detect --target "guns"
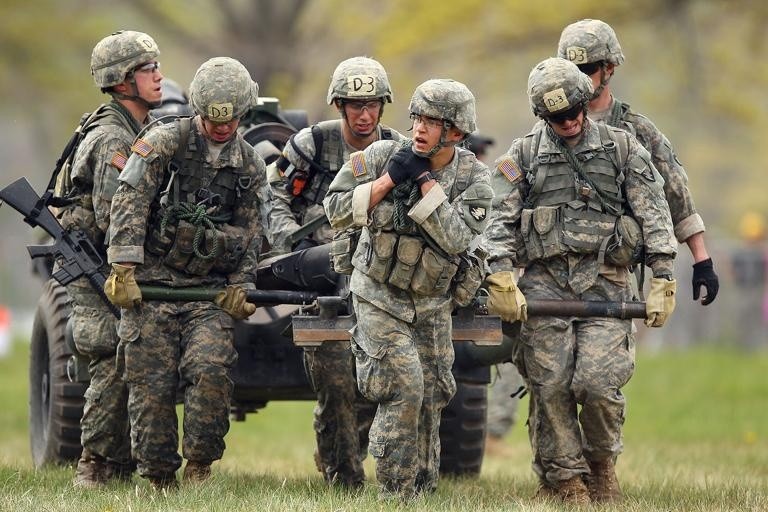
[1,177,120,320]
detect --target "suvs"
[28,77,517,487]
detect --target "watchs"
[657,274,674,281]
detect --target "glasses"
[580,63,606,75]
[547,106,581,124]
[134,61,162,75]
[411,115,444,130]
[344,99,381,113]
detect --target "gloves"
[103,265,142,310]
[213,285,256,320]
[645,276,677,329]
[692,259,720,307]
[387,145,430,185]
[485,271,527,322]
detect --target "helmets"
[327,58,393,104]
[189,57,260,122]
[526,58,592,119]
[558,19,625,67]
[91,29,159,89]
[410,79,477,134]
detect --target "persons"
[482,56,676,510]
[466,117,522,463]
[259,55,411,497]
[52,29,165,491]
[321,77,496,504]
[104,55,267,492]
[525,17,719,504]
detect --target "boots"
[150,459,212,488]
[532,460,620,505]
[75,446,135,490]
